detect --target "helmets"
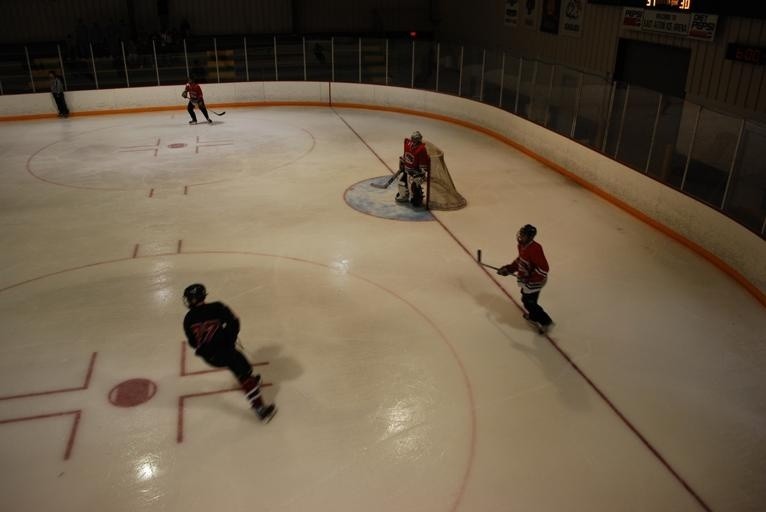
[183,284,206,308]
[411,131,422,141]
[516,224,536,244]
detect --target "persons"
[47,69,69,117]
[497,224,556,334]
[183,284,275,421]
[182,72,212,126]
[395,130,432,208]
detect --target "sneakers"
[189,118,212,123]
[256,404,274,420]
[523,313,553,334]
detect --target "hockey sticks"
[370,170,403,188]
[477,249,518,276]
[186,97,226,116]
